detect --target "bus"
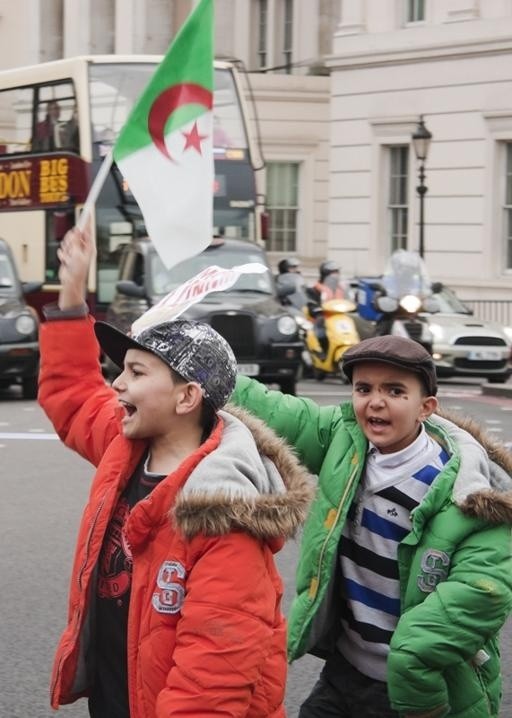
[0,53,269,313]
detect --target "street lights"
[410,115,432,259]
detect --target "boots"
[318,337,328,354]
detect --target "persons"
[229,335,512,718]
[371,252,436,338]
[36,207,318,718]
[277,257,302,273]
[305,262,352,356]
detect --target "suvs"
[109,237,308,397]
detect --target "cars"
[362,274,512,385]
[1,239,45,400]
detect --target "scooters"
[301,273,369,386]
[374,250,440,356]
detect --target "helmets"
[278,258,301,274]
[319,260,339,282]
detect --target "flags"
[111,0,217,270]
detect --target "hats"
[341,335,437,397]
[93,319,238,414]
